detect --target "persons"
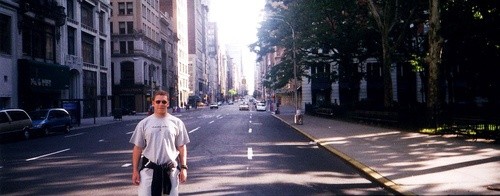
[129,90,190,196]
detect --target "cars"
[210,103,218,109]
[0,109,33,139]
[228,97,267,111]
[31,108,74,136]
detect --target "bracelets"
[181,165,188,169]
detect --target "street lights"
[267,17,299,110]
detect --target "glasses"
[155,100,167,104]
